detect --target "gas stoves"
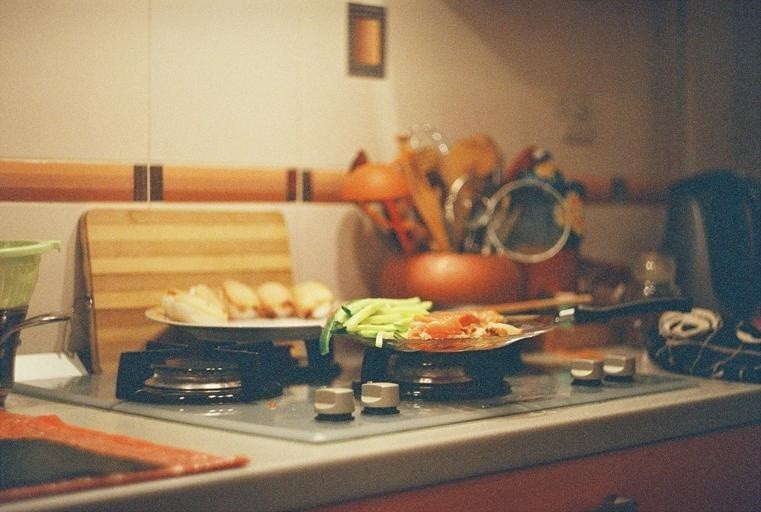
[1,304,696,444]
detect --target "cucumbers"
[317,295,433,356]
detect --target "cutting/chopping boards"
[75,198,311,375]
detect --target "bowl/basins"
[2,235,63,305]
[374,245,577,313]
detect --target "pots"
[339,318,562,353]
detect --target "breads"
[161,279,336,326]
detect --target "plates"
[147,301,326,345]
